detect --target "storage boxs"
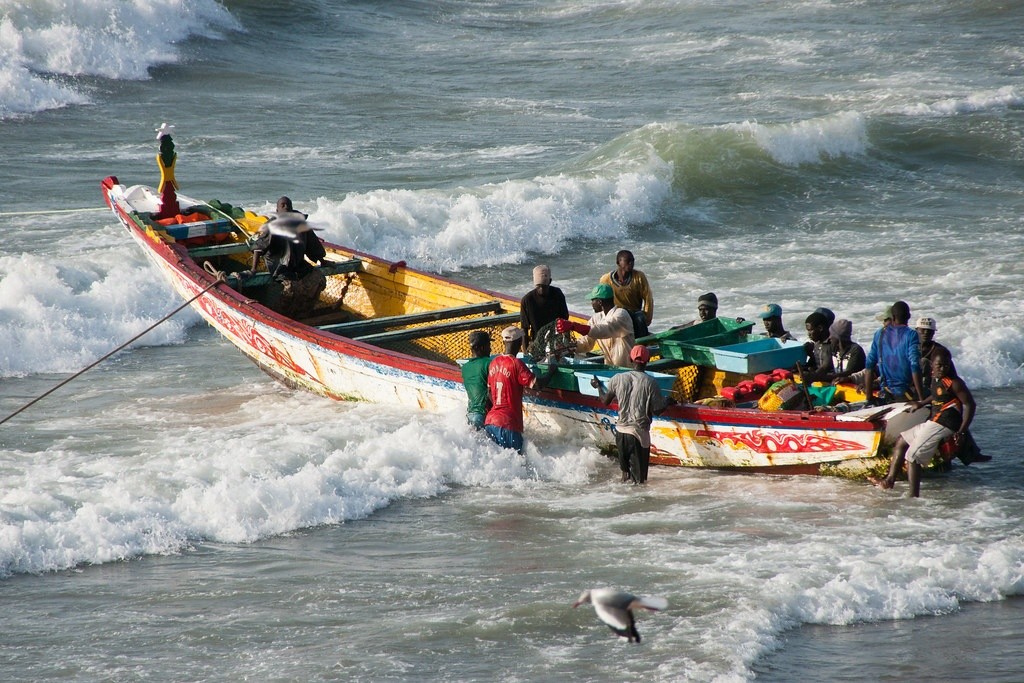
[658,315,756,360]
[536,362,628,391]
[709,337,807,374]
[676,333,767,367]
[574,370,676,400]
[455,352,532,369]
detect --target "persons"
[487,326,557,454]
[669,292,719,329]
[599,249,653,339]
[247,195,336,315]
[880,346,977,495]
[832,300,992,466]
[520,264,569,354]
[557,283,635,367]
[736,303,796,344]
[590,345,678,483]
[789,307,866,386]
[462,330,495,431]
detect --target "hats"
[584,283,615,299]
[914,317,938,331]
[829,319,852,340]
[502,326,525,341]
[875,304,894,322]
[756,303,782,318]
[631,345,649,363]
[533,265,551,286]
[697,293,718,309]
[469,330,496,344]
[815,308,835,326]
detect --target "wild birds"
[570,589,669,645]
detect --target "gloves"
[556,319,591,336]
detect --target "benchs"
[185,236,524,345]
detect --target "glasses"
[883,317,889,322]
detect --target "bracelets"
[957,432,965,436]
